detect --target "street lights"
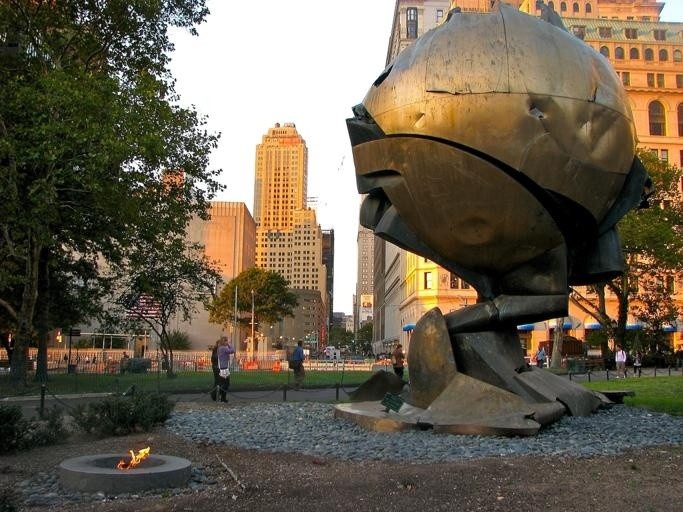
[250,289,254,363]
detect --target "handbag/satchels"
[219,368,230,378]
[289,360,302,369]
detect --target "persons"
[532,346,539,367]
[210,341,219,399]
[632,349,643,377]
[215,335,235,401]
[674,344,682,371]
[4,332,14,370]
[291,339,305,393]
[614,344,627,379]
[392,343,404,379]
[537,346,545,368]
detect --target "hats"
[396,344,402,349]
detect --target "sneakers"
[211,394,228,402]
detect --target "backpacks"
[391,355,397,364]
